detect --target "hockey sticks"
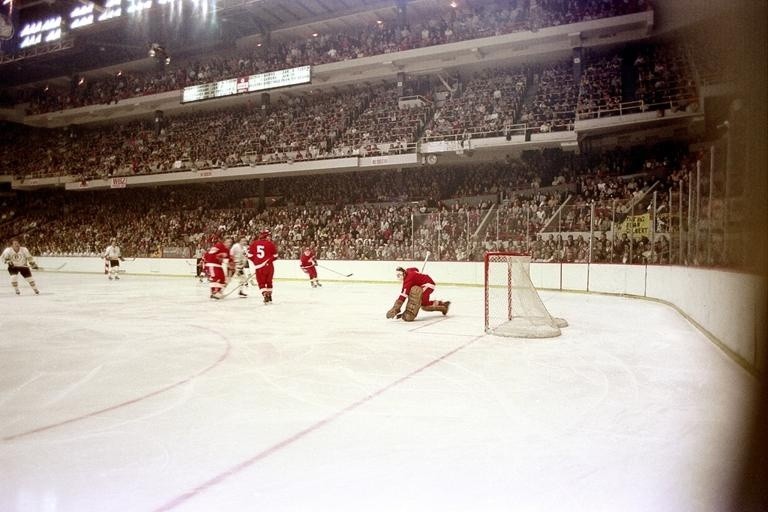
[210,274,255,301]
[318,265,352,277]
[39,263,67,270]
[125,257,136,261]
[397,251,432,319]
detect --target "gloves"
[386,300,404,319]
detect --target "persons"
[0,237,41,297]
[385,265,451,321]
[242,231,279,304]
[221,236,252,296]
[1,0,766,288]
[202,237,234,299]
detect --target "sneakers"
[239,290,272,303]
[442,300,451,316]
[15,289,41,295]
